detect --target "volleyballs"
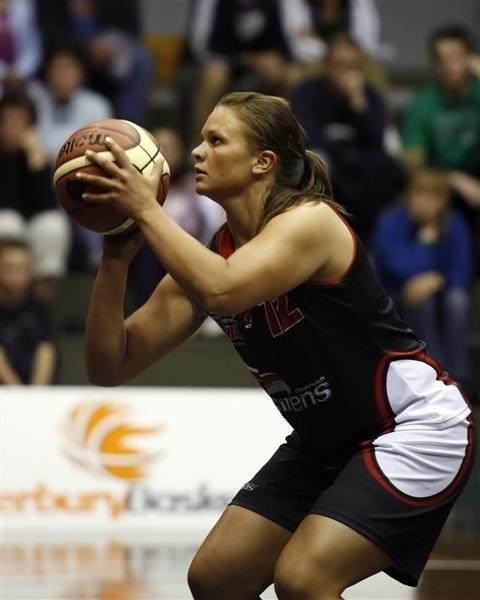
[53,120,170,236]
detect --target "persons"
[1,1,479,386]
[76,91,476,600]
